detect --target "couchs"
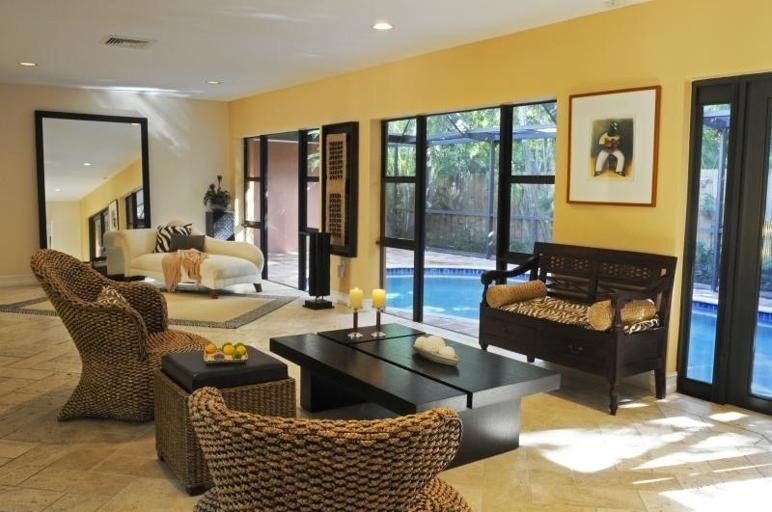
[114,219,266,300]
[101,231,126,278]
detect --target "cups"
[416,333,456,357]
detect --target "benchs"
[479,240,678,416]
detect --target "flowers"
[203,171,233,206]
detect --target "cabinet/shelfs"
[205,211,235,240]
[301,229,334,310]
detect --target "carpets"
[158,288,301,329]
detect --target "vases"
[212,203,228,212]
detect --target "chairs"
[186,384,471,511]
[31,247,211,427]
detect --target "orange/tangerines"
[207,342,247,359]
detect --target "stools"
[151,345,295,495]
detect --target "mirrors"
[36,108,152,285]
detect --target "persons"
[594,120,627,177]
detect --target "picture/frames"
[109,198,119,231]
[322,116,362,258]
[564,83,663,209]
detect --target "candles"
[373,289,386,309]
[350,286,363,310]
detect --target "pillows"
[152,222,193,254]
[486,279,551,309]
[170,233,206,253]
[585,294,657,332]
